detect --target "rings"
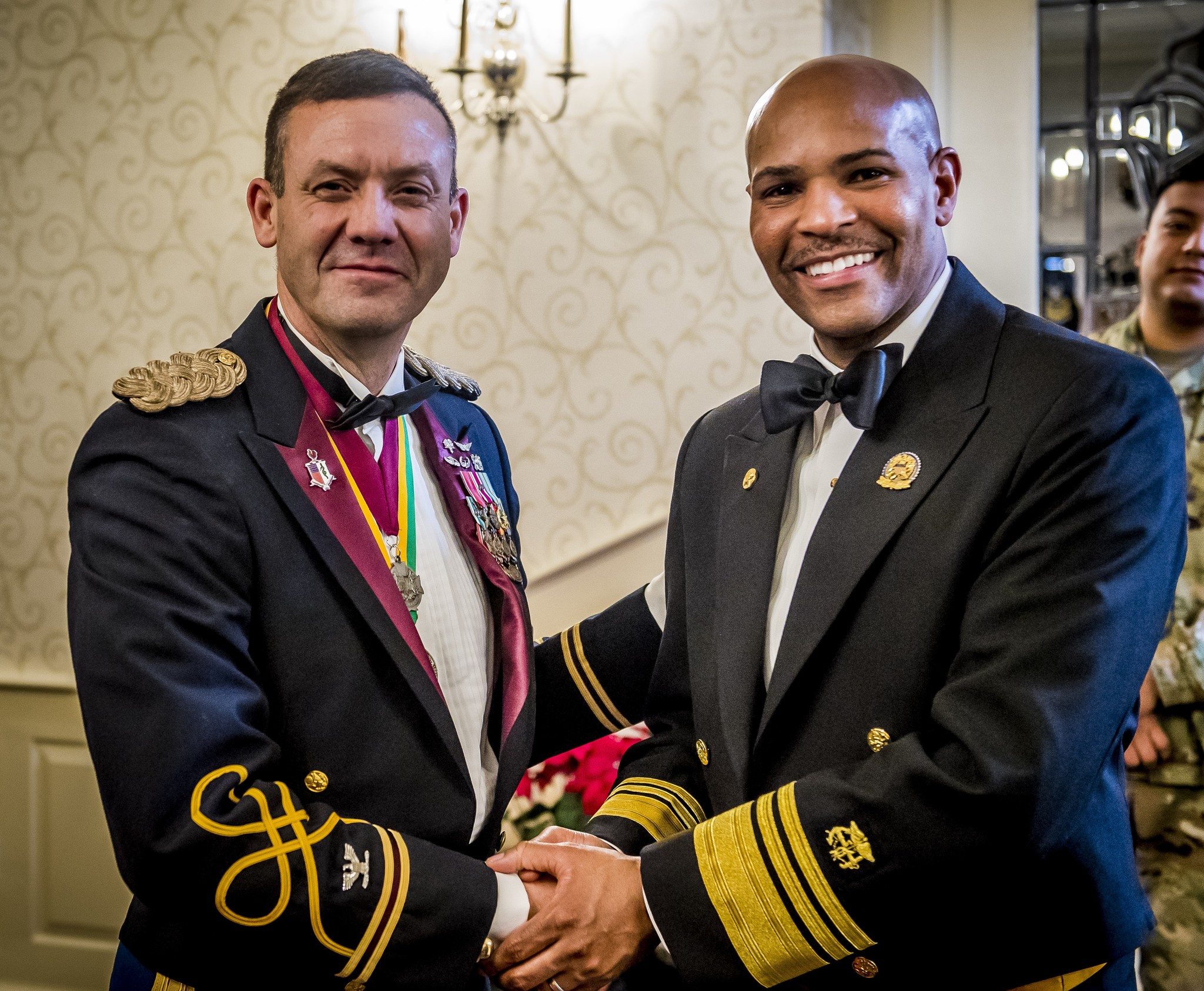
[546,977,565,991]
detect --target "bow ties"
[275,309,441,431]
[759,342,905,436]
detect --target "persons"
[66,47,682,991]
[1082,158,1202,991]
[481,55,1187,991]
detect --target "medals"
[391,560,424,613]
